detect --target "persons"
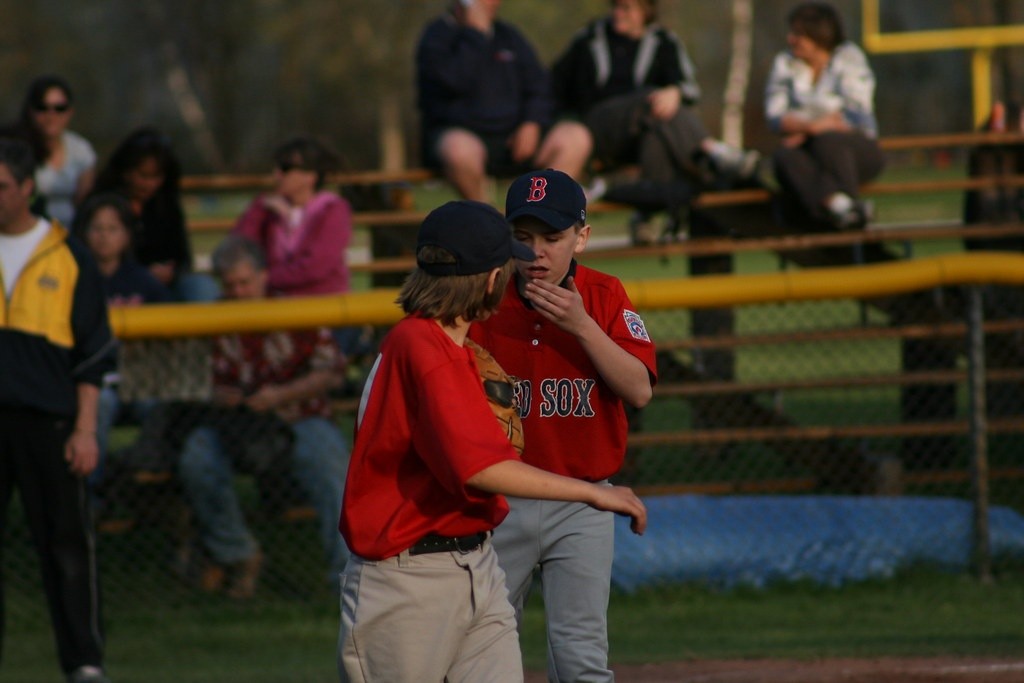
[763,1,888,233]
[0,77,427,602]
[339,198,647,683]
[463,168,657,683]
[0,139,121,683]
[544,0,706,211]
[414,0,545,208]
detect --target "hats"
[506,169,586,230]
[416,200,536,277]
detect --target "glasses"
[35,103,67,113]
[278,161,302,172]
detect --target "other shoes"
[66,665,108,683]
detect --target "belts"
[408,530,493,555]
